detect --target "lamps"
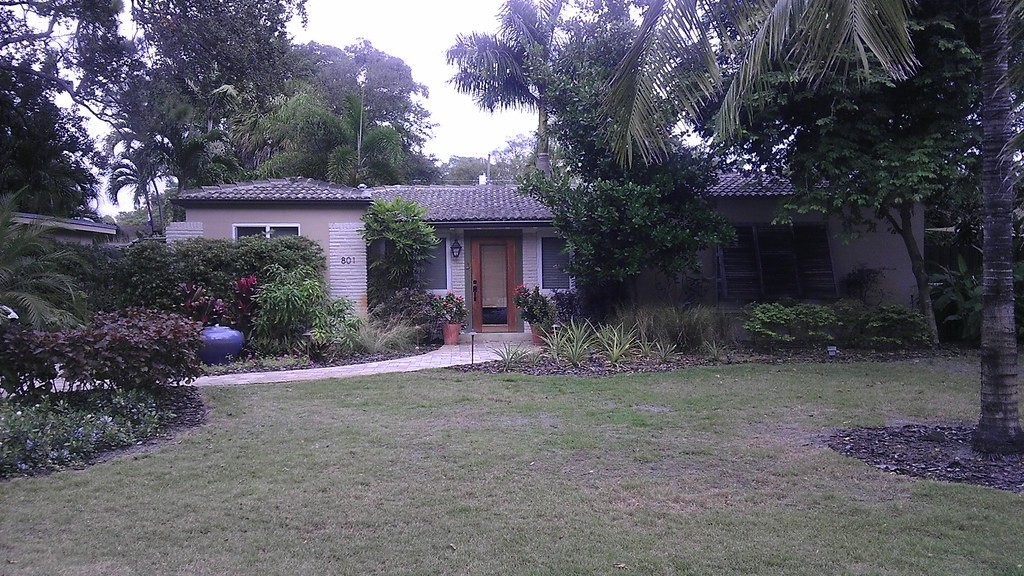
[450,239,462,257]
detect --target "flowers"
[512,283,553,323]
[433,290,468,324]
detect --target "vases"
[529,324,552,346]
[197,326,243,366]
[442,324,461,345]
